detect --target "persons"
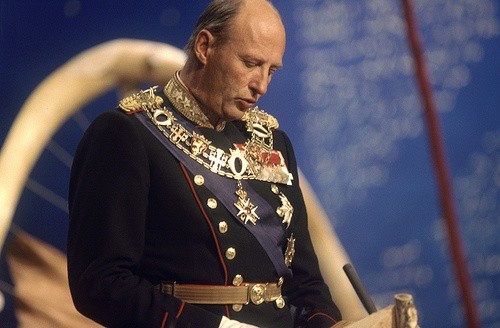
[66,0,346,328]
